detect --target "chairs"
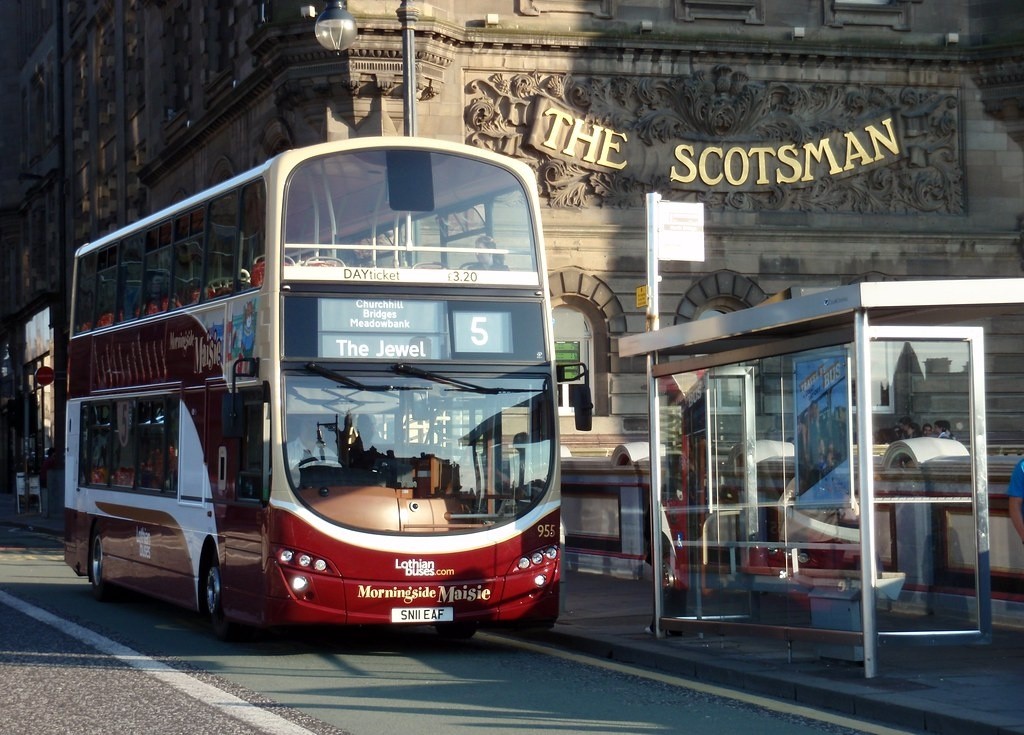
[87,436,269,496]
[77,254,510,333]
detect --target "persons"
[495,432,536,497]
[41,447,60,485]
[873,411,958,445]
[276,415,342,487]
[796,401,845,483]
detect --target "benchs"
[671,531,908,618]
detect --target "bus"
[61,135,595,643]
